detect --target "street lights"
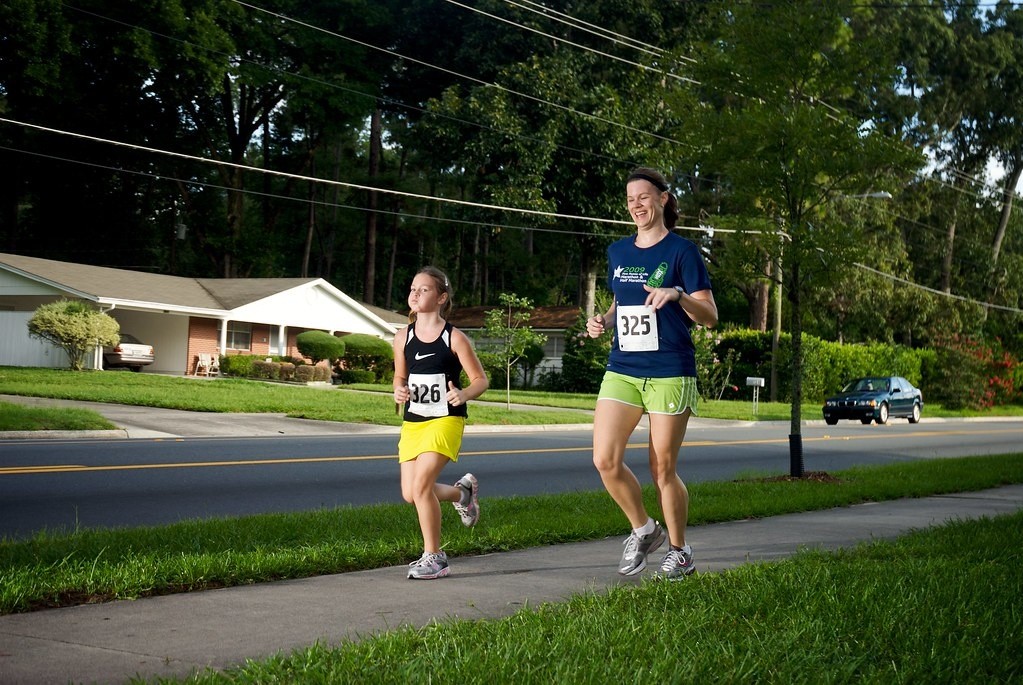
[770,191,893,401]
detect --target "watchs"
[672,285,684,302]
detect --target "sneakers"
[452,473,479,528]
[406,551,451,579]
[618,518,666,576]
[651,545,696,583]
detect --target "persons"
[393,266,490,579]
[586,169,718,584]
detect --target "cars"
[822,377,924,425]
[103,334,155,372]
